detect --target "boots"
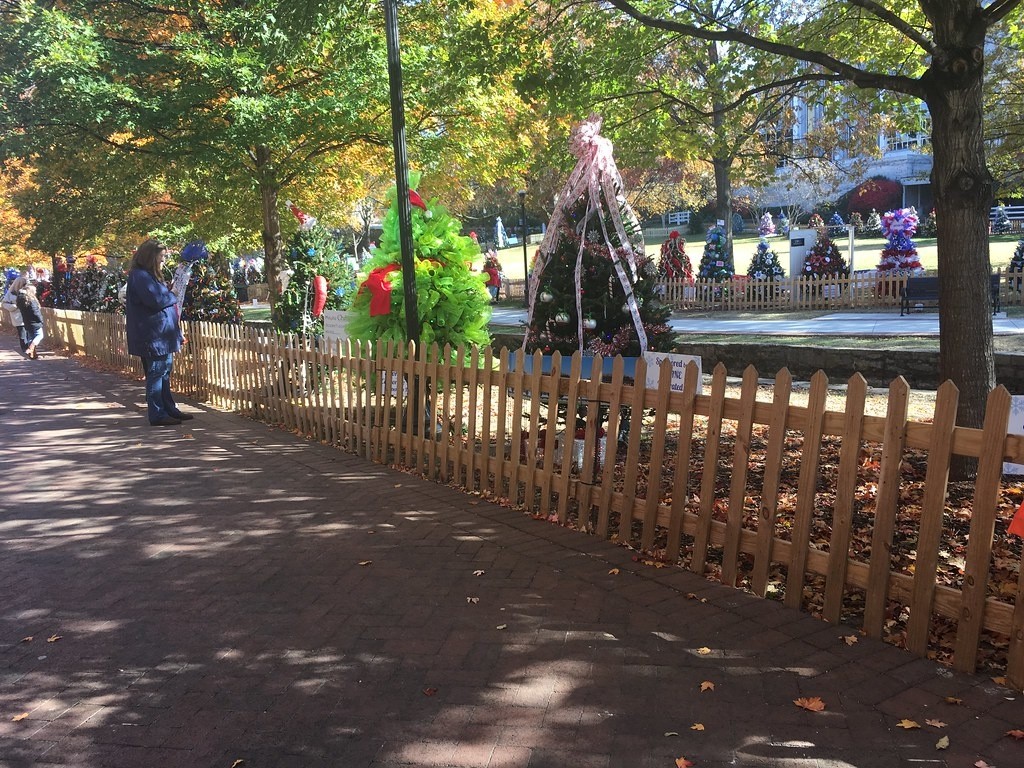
[24,343,38,360]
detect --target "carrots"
[313,276,327,317]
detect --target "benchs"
[901,273,1001,316]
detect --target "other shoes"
[172,412,193,420]
[150,416,182,426]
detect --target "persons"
[482,261,501,305]
[125,240,192,426]
[2,270,44,359]
